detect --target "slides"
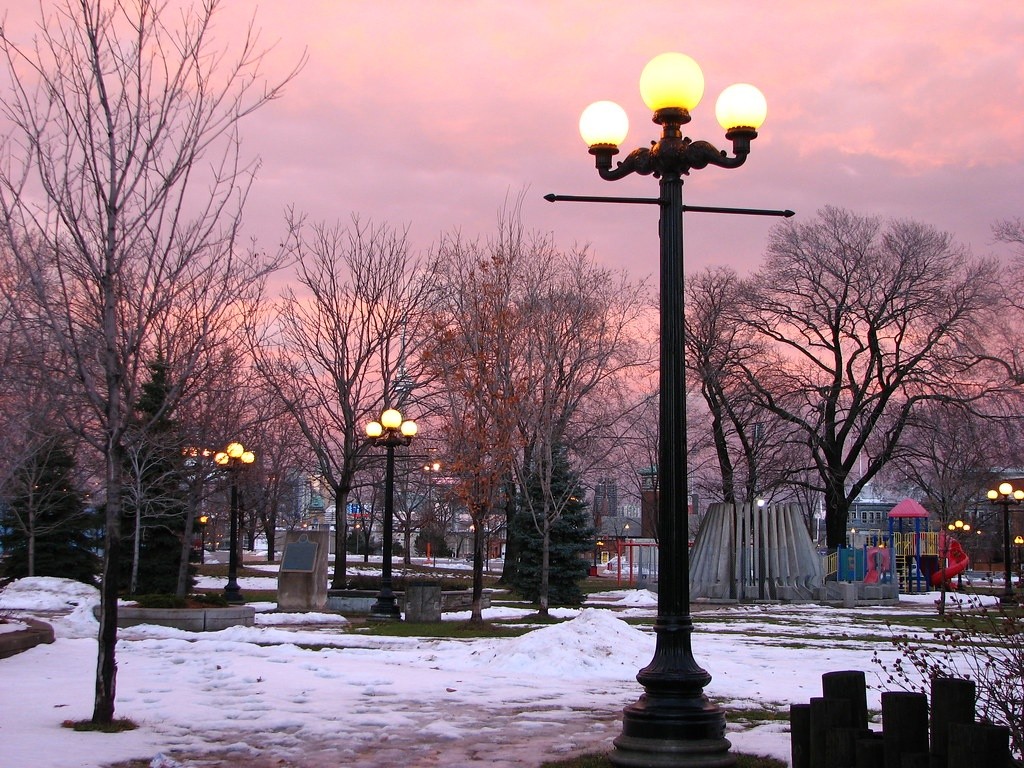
[864,549,890,583]
[931,530,970,589]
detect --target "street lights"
[986,482,1024,607]
[596,542,603,564]
[213,443,255,605]
[345,411,431,620]
[539,52,797,765]
[948,521,970,592]
[1014,535,1024,587]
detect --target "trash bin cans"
[608,563,612,570]
[403,581,442,622]
